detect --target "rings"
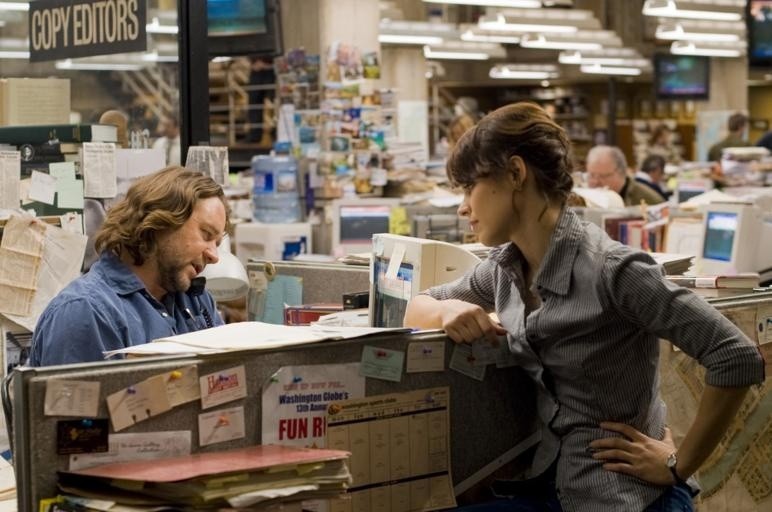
[181,308,197,323]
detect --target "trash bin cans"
[664,450,682,486]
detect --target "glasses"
[0,35,32,59]
[56,53,144,72]
[641,0,745,60]
[146,14,181,33]
[378,0,649,79]
[145,44,228,63]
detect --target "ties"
[369,232,484,329]
[652,51,710,101]
[694,203,771,275]
[676,178,714,206]
[745,2,772,72]
[332,198,398,262]
[205,1,279,55]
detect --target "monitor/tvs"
[54,443,353,512]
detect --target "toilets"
[185,277,206,296]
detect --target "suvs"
[251,156,302,225]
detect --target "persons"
[757,133,772,152]
[403,102,765,511]
[585,144,665,206]
[708,114,754,160]
[30,168,229,365]
[449,96,486,147]
[99,110,129,147]
[639,155,674,202]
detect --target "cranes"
[56,443,353,507]
